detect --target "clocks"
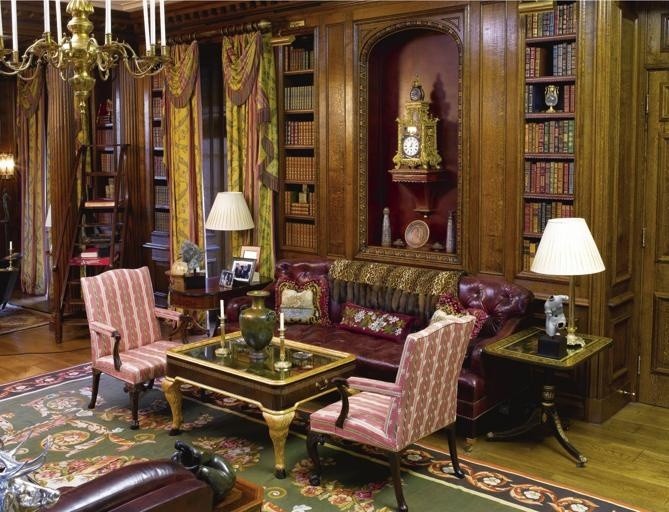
[391,71,444,171]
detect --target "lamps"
[0,0,172,146]
[0,152,15,179]
[530,217,606,346]
[205,191,256,268]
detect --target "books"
[284,45,317,252]
[518,1,579,272]
[71,63,169,267]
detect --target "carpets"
[1,362,653,512]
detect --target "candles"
[280,313,285,329]
[9,241,13,249]
[219,299,225,317]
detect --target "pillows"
[435,292,487,361]
[274,274,332,328]
[337,301,414,343]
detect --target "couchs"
[220,259,534,453]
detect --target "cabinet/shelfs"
[280,28,319,255]
[150,52,171,235]
[517,0,580,277]
[91,65,119,257]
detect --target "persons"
[410,227,419,243]
[222,262,250,286]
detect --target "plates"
[292,350,314,360]
[236,338,245,346]
[403,220,430,249]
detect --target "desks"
[486,326,616,473]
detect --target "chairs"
[308,316,477,511]
[80,266,186,430]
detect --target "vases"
[239,290,277,360]
